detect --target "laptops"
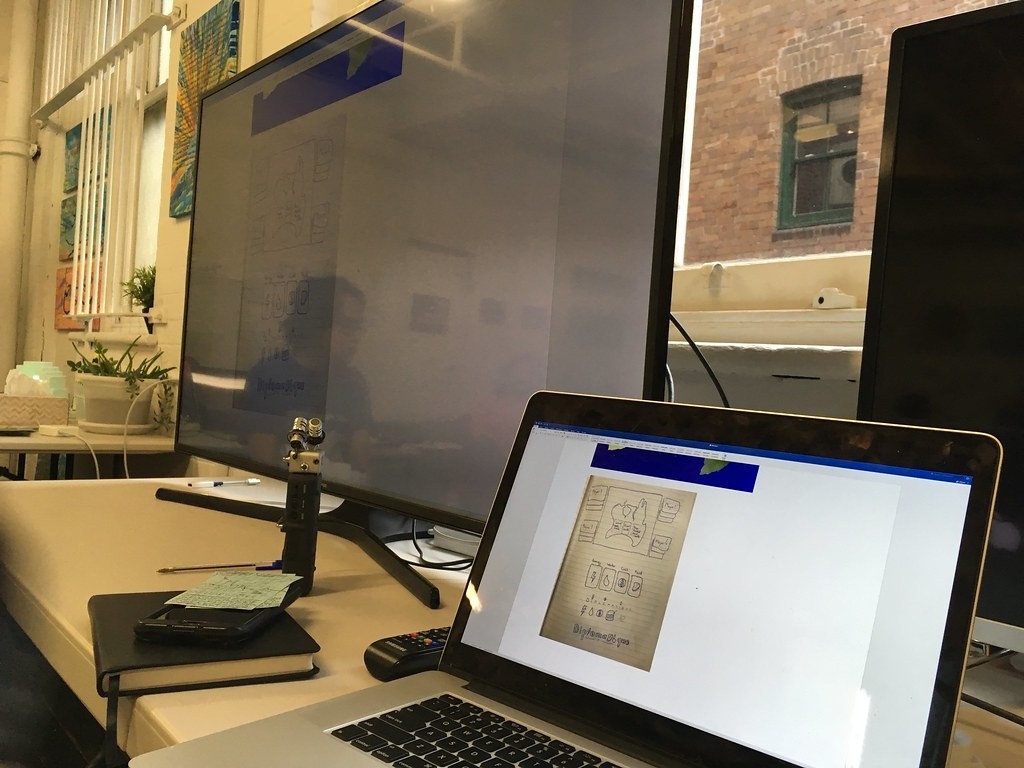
[128,391,1003,768]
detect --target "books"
[88,590,320,696]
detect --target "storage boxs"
[1,396,69,426]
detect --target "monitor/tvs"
[854,0,1024,728]
[156,0,705,608]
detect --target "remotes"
[364,626,451,683]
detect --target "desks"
[0,479,1024,768]
[0,427,175,481]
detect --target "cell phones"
[135,585,303,645]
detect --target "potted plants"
[66,334,175,433]
[120,261,157,334]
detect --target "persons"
[242,276,373,475]
